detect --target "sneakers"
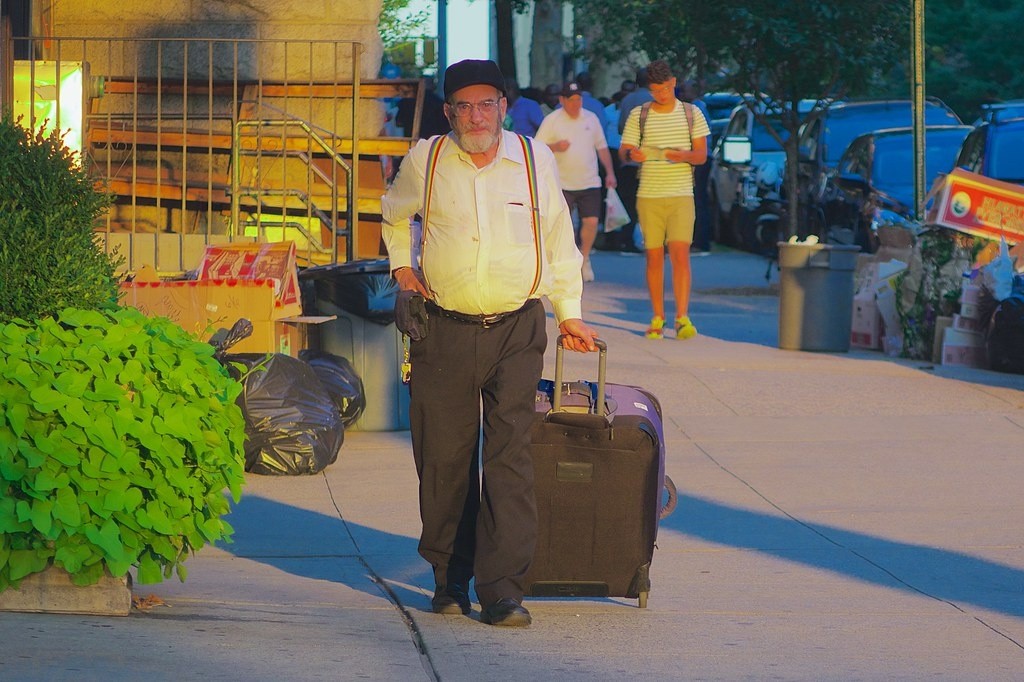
[674,315,697,339]
[645,316,666,341]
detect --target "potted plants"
[0,104,278,615]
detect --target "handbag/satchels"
[605,185,630,232]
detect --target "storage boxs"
[121,240,339,354]
[852,167,1024,370]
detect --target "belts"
[424,299,542,330]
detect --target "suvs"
[707,99,842,252]
[962,104,1024,186]
[782,96,966,202]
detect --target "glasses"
[650,82,674,98]
[447,96,502,118]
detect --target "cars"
[840,123,974,226]
[700,91,775,149]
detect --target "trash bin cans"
[298,257,410,431]
[776,241,862,354]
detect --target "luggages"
[526,332,676,606]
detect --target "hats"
[444,58,505,104]
[562,82,582,96]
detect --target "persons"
[676,79,712,257]
[535,82,617,282]
[576,69,652,255]
[386,75,452,183]
[381,60,600,627]
[618,61,711,339]
[504,79,561,137]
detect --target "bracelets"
[625,150,631,162]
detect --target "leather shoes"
[431,590,468,616]
[482,597,531,629]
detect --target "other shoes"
[581,261,595,283]
[620,244,642,258]
[691,244,711,257]
